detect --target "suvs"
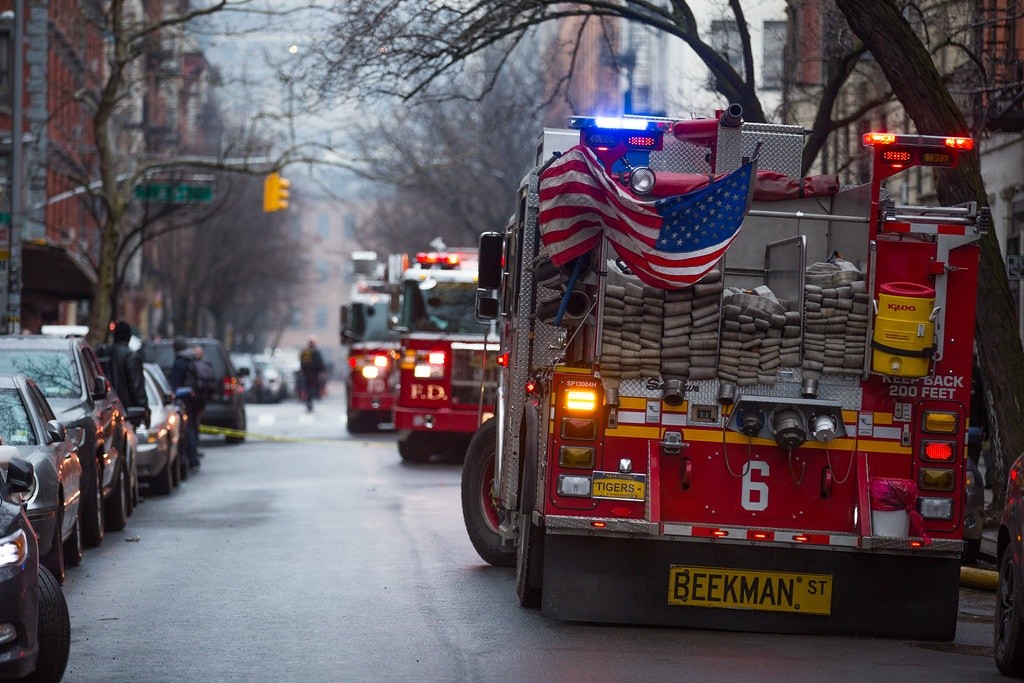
[143,336,251,445]
[0,334,136,549]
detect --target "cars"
[98,357,194,498]
[228,350,301,404]
[0,373,83,585]
[0,456,72,683]
[993,453,1024,681]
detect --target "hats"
[172,339,188,349]
[114,321,132,343]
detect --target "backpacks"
[184,361,216,403]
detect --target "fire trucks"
[388,241,502,465]
[461,99,991,640]
[340,251,396,436]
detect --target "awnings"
[22,238,100,299]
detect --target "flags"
[537,145,754,292]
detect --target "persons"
[168,336,208,467]
[301,339,324,410]
[98,321,152,432]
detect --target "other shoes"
[195,451,204,456]
[189,459,199,468]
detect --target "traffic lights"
[263,173,290,213]
